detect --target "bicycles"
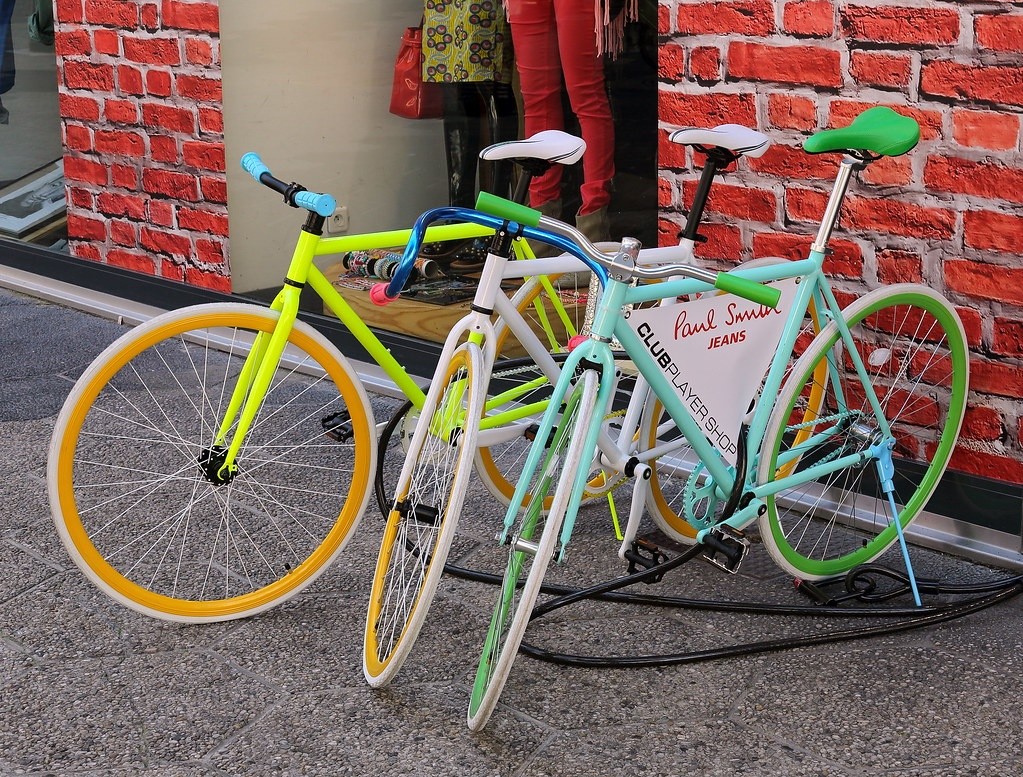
[44,131,665,626]
[467,104,972,733]
[362,119,841,691]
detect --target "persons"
[501,0,639,291]
[419,0,521,273]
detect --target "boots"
[526,198,564,259]
[552,205,615,288]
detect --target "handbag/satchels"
[389,14,443,119]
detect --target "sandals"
[417,223,473,259]
[450,236,512,269]
